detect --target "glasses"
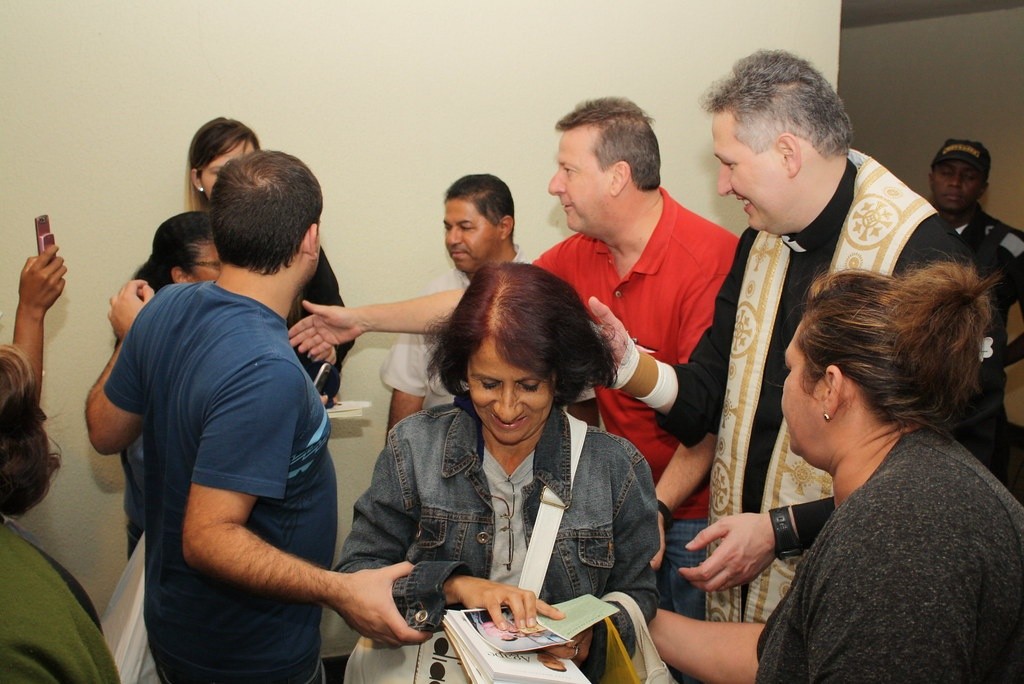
[481,474,514,572]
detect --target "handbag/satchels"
[600,592,678,684]
[344,629,471,684]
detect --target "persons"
[591,48,970,684]
[477,608,545,642]
[83,147,433,684]
[649,256,1024,684]
[12,243,66,404]
[289,97,741,684]
[99,209,222,684]
[380,172,598,451]
[332,259,659,684]
[185,117,356,409]
[923,131,1024,485]
[0,342,124,683]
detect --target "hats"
[930,138,991,182]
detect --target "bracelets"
[768,505,804,561]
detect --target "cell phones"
[314,363,332,393]
[35,215,56,266]
